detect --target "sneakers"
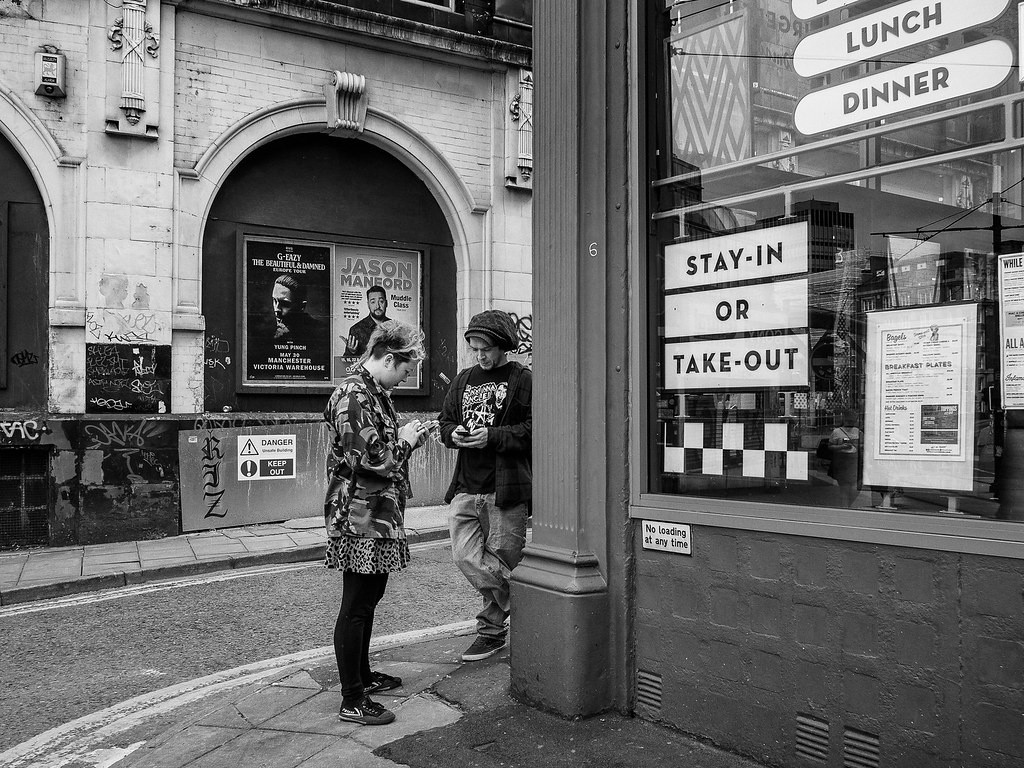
[461,636,507,661]
[364,671,402,696]
[338,697,396,725]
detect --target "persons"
[437,311,532,660]
[828,408,864,507]
[339,286,392,356]
[271,274,323,337]
[323,319,432,725]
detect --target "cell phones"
[454,430,469,436]
[417,423,436,432]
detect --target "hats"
[464,310,519,352]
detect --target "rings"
[455,441,459,444]
